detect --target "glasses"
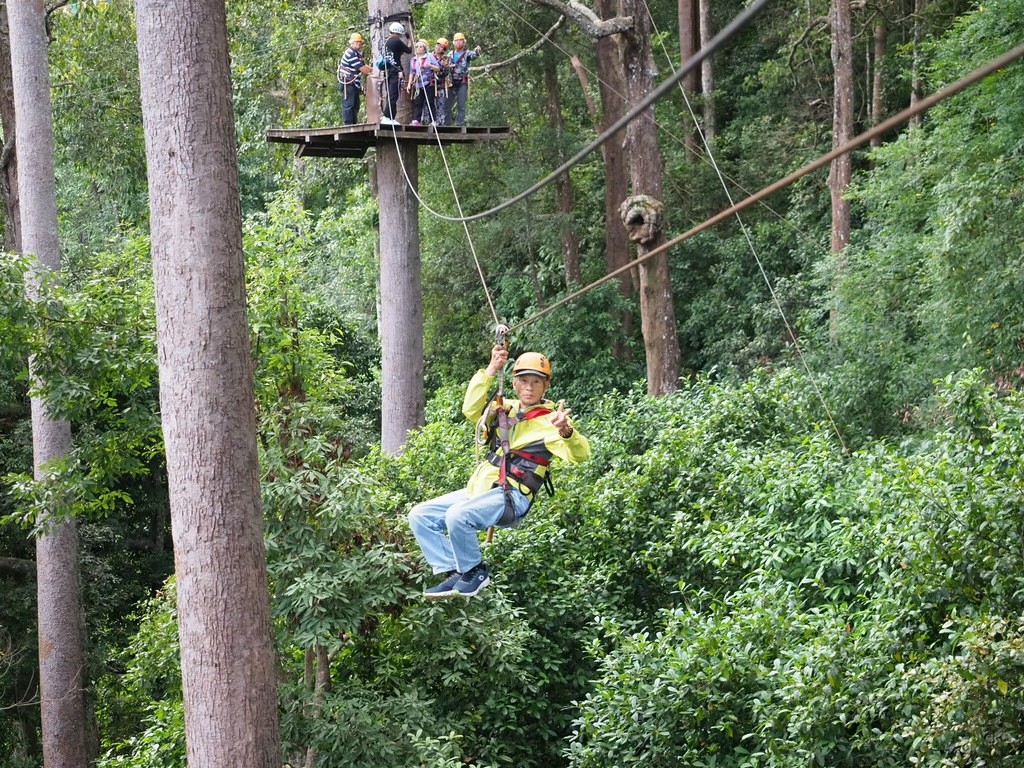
[415,46,423,48]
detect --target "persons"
[336,34,373,126]
[406,338,591,599]
[374,22,483,127]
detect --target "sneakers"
[452,564,490,597]
[409,119,421,125]
[380,116,400,125]
[423,573,462,597]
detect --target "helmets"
[437,38,448,48]
[349,33,362,41]
[453,33,465,41]
[513,352,551,378]
[419,39,430,47]
[389,22,405,35]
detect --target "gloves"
[404,32,410,40]
